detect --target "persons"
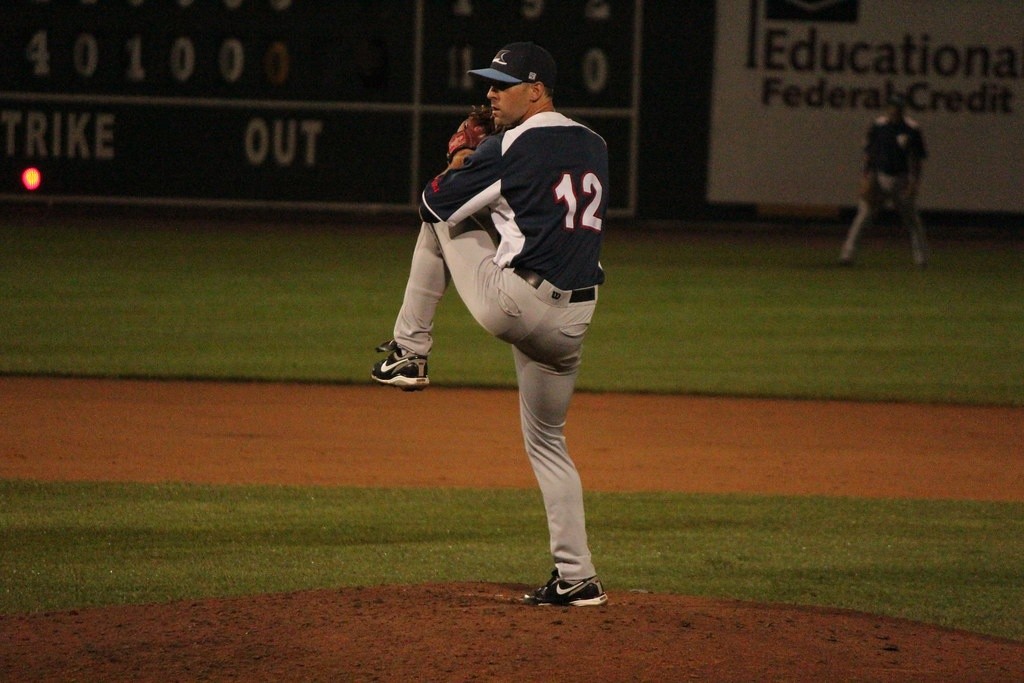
[369,49,613,609]
[838,92,928,271]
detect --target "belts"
[510,266,599,305]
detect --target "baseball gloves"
[446,105,494,161]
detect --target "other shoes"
[825,255,856,268]
[907,262,928,272]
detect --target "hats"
[885,93,907,108]
[466,41,559,88]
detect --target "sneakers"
[370,340,429,391]
[519,570,609,608]
